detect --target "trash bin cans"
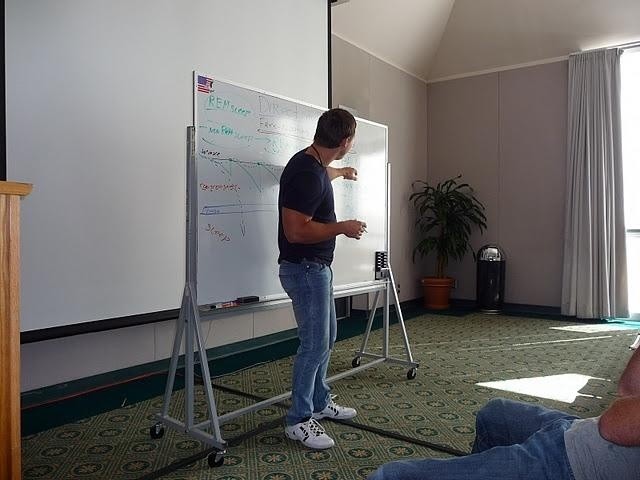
[475,244,506,316]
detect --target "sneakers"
[311,399,357,419]
[284,420,334,449]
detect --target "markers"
[354,218,368,234]
[211,300,234,309]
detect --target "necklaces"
[310,144,323,166]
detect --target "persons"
[368,342,640,480]
[277,107,368,449]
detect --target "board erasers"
[237,295,259,304]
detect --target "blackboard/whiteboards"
[191,70,389,318]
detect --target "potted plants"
[406,171,489,310]
